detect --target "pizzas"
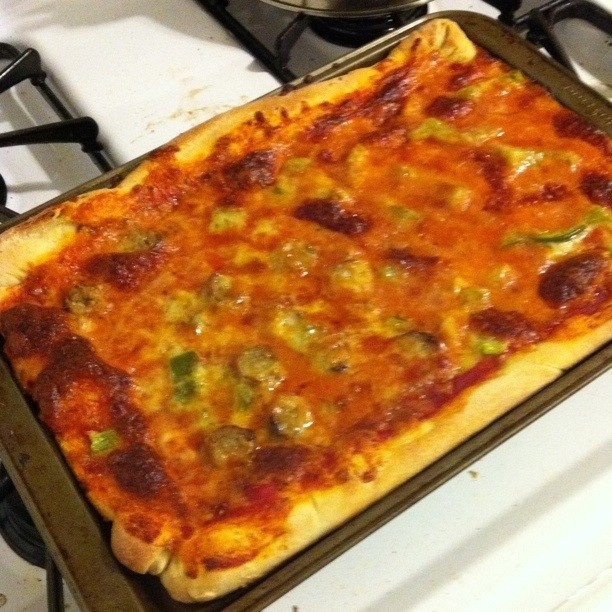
[0,18,612,605]
